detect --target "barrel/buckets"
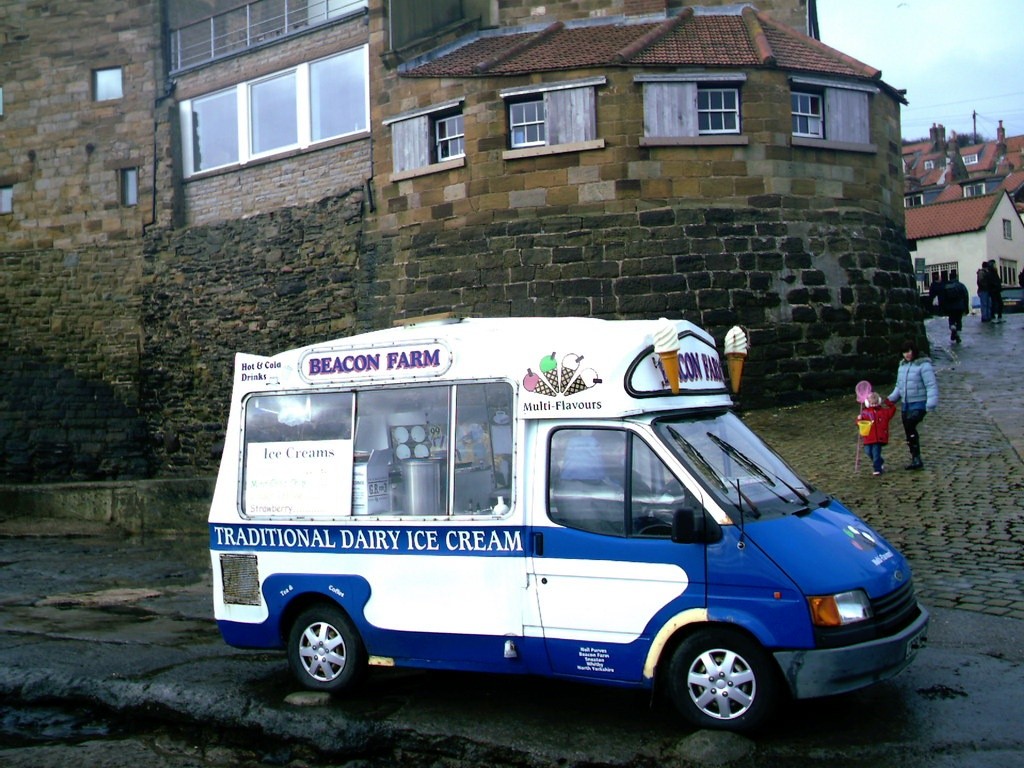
[401,458,440,514]
[388,411,430,462]
[858,420,871,435]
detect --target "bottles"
[493,496,509,516]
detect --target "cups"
[496,411,505,421]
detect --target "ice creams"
[723,325,748,393]
[653,317,681,394]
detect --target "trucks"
[205,314,930,732]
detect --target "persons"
[855,392,897,475]
[558,431,649,533]
[977,260,1006,324]
[888,341,939,469]
[929,273,969,342]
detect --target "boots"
[905,458,915,469]
[911,456,924,470]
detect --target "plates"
[494,415,510,424]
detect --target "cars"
[1000,286,1024,313]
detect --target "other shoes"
[881,465,883,469]
[956,335,962,343]
[873,471,880,476]
[990,318,999,324]
[997,318,1007,323]
[951,329,957,341]
